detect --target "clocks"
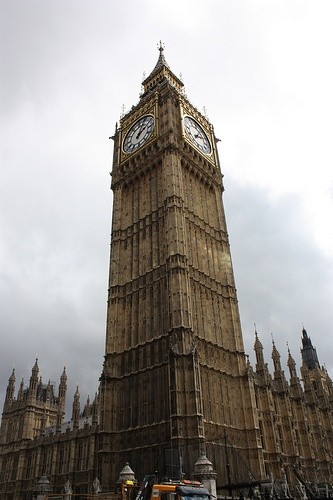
[121,113,155,155]
[183,114,214,158]
